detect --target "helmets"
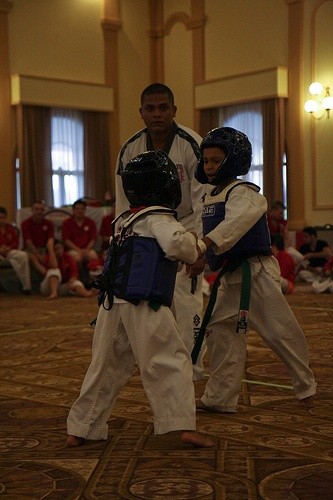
[121,150,182,210]
[194,127,252,185]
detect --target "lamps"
[304,82,333,119]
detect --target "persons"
[180,126,320,414]
[0,199,116,300]
[114,82,206,383]
[267,201,333,292]
[66,151,216,449]
